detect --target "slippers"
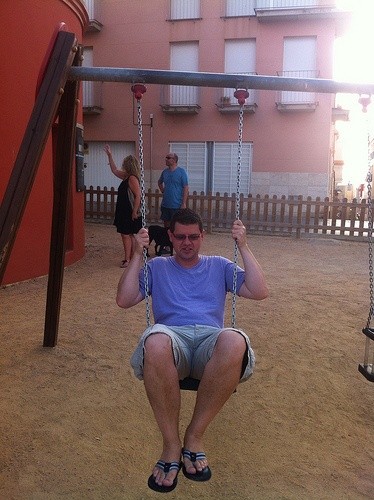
[147,460,183,492]
[181,447,212,481]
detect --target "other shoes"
[156,251,161,256]
[120,259,129,268]
[144,253,150,258]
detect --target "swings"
[357,91,374,383]
[131,80,249,393]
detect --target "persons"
[157,154,189,254]
[101,144,142,268]
[113,214,269,492]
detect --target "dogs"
[145,225,174,257]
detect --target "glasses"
[170,230,201,241]
[165,156,175,159]
